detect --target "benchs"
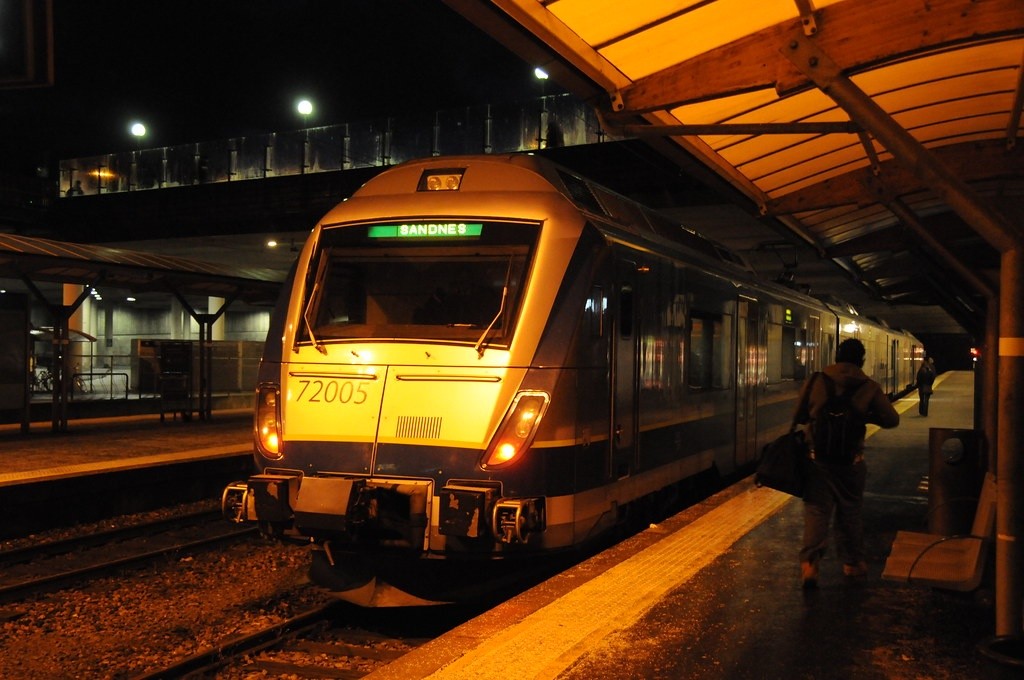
[879,471,997,636]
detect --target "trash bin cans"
[928,427,982,537]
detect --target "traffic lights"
[972,349,978,362]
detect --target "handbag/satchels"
[757,372,837,503]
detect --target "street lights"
[131,123,146,151]
[298,99,312,127]
[535,67,548,97]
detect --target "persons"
[545,120,565,148]
[916,357,935,416]
[793,339,900,589]
[197,155,210,183]
[66,180,83,195]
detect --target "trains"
[222,153,927,613]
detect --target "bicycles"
[36,362,87,395]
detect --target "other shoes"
[842,561,871,601]
[799,558,822,592]
